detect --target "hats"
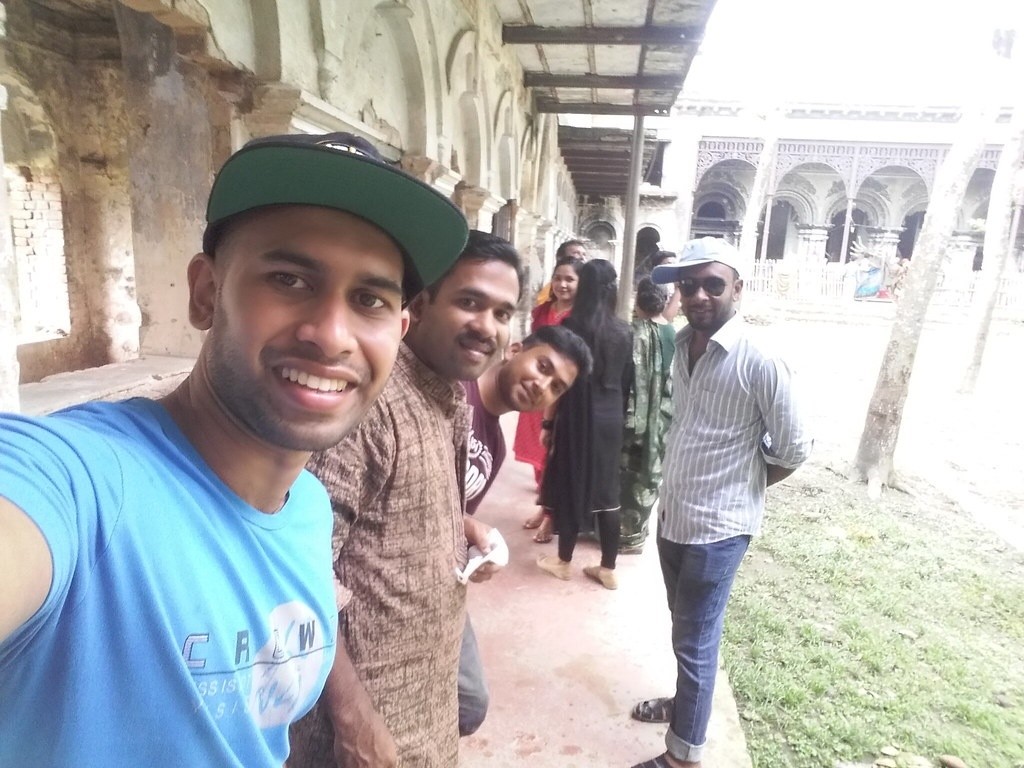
[206,131,469,308]
[651,236,741,284]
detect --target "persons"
[0,132,593,768]
[632,236,813,768]
[512,238,681,590]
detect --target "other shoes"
[631,691,673,722]
[616,544,643,555]
[582,566,617,590]
[630,750,703,768]
[536,551,572,580]
[534,526,553,543]
[522,515,542,528]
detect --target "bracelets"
[541,419,552,430]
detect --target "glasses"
[678,277,733,297]
